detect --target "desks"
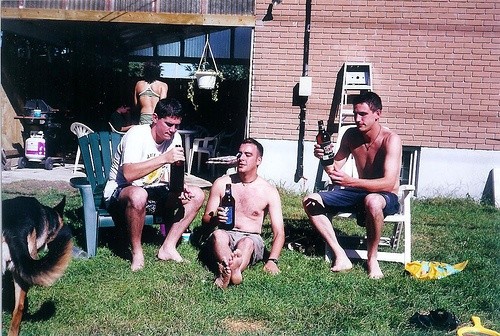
[178,129,200,175]
[14,116,46,139]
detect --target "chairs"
[70,121,112,174]
[67,130,174,259]
[319,123,414,267]
[108,120,127,135]
[188,135,220,176]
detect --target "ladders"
[335,62,373,188]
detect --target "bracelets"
[267,258,278,265]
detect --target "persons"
[134,59,168,125]
[302,91,403,280]
[200,138,285,289]
[102,98,205,271]
[109,101,135,132]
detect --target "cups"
[34,110,42,117]
[181,233,191,245]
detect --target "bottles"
[221,184,236,230]
[169,145,185,193]
[316,120,335,167]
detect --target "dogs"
[1,194,73,336]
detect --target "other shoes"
[409,309,458,330]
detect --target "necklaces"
[239,174,258,186]
[364,126,382,151]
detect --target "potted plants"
[183,60,226,105]
